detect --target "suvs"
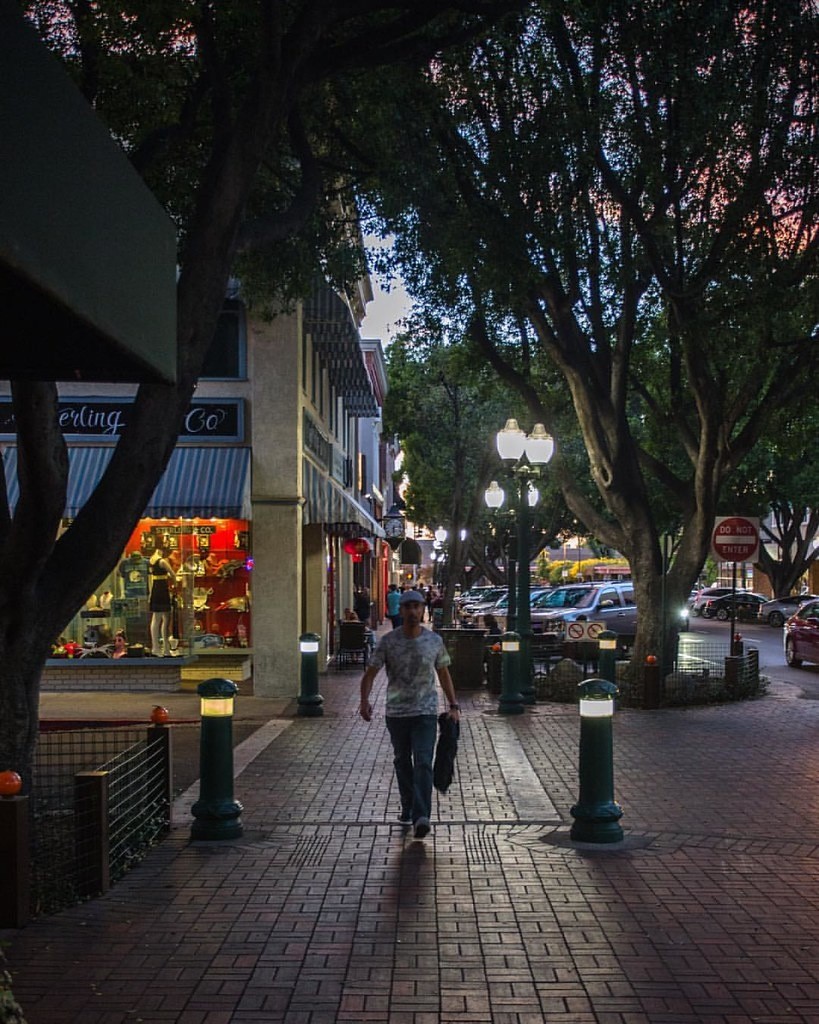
[529,581,637,656]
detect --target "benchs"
[486,644,599,677]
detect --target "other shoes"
[399,818,413,826]
[414,817,430,839]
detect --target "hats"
[399,592,426,605]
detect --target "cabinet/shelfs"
[144,547,252,654]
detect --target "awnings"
[310,277,379,419]
[2,444,252,521]
[400,537,421,564]
[302,459,386,540]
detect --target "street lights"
[484,480,539,706]
[495,419,555,716]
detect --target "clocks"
[384,517,405,538]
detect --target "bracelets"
[449,703,461,710]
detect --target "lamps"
[501,631,521,651]
[578,678,619,717]
[299,632,321,652]
[598,630,617,649]
[197,678,239,716]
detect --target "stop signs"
[712,517,760,562]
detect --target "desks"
[364,631,373,637]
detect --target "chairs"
[336,621,367,672]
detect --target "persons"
[386,582,444,629]
[51,631,127,659]
[483,614,502,635]
[344,608,376,662]
[148,537,177,659]
[359,591,462,839]
[456,611,468,624]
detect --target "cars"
[757,594,819,628]
[688,590,697,604]
[704,593,768,621]
[693,587,748,619]
[454,585,548,634]
[783,600,819,668]
